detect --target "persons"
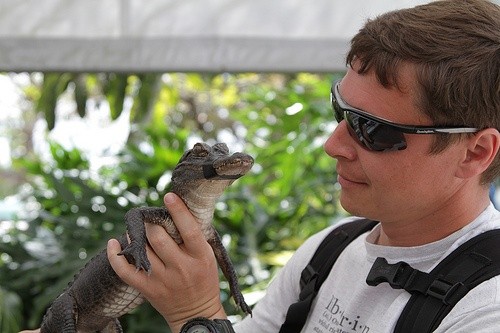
[107,0,500,333]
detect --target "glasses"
[331,81,484,152]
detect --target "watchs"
[179,316,235,333]
